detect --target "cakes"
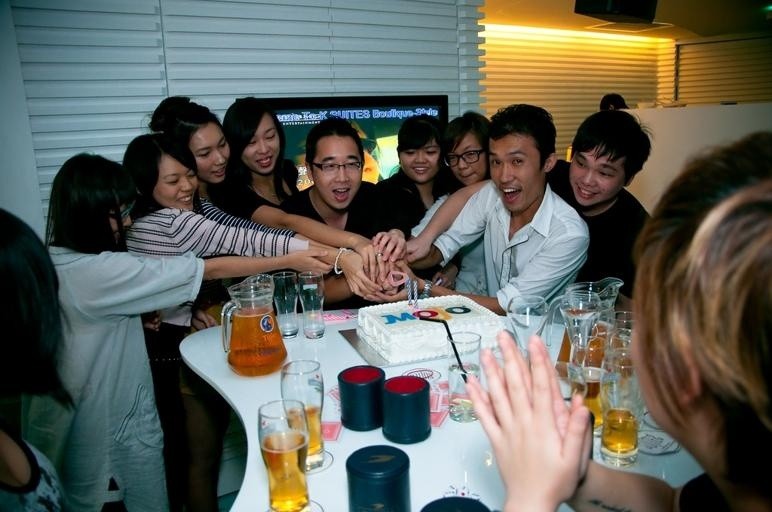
[355,293,513,367]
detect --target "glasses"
[312,160,362,173]
[443,149,485,167]
[110,201,134,220]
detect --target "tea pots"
[545,277,634,388]
[221,274,288,377]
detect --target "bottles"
[346,446,410,511]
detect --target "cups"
[257,360,333,512]
[446,331,481,421]
[337,366,431,444]
[507,290,637,469]
[272,270,324,340]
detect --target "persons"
[0,93,655,512]
[464,130,772,512]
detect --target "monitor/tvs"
[235,94,448,193]
